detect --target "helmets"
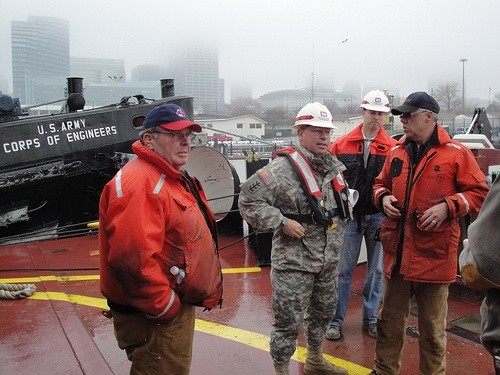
[292,102,335,130]
[360,90,392,114]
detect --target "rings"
[432,220,437,225]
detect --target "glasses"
[399,110,428,118]
[149,131,196,143]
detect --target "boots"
[274,361,290,375]
[303,341,351,374]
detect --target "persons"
[98,104,224,374]
[324,88,400,340]
[237,101,355,375]
[370,92,491,375]
[458,170,500,374]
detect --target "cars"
[452,126,500,185]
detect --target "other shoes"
[326,321,342,341]
[361,318,377,338]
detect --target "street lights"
[459,57,467,114]
[107,74,123,102]
[389,94,394,130]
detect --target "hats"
[143,104,202,132]
[391,91,440,115]
